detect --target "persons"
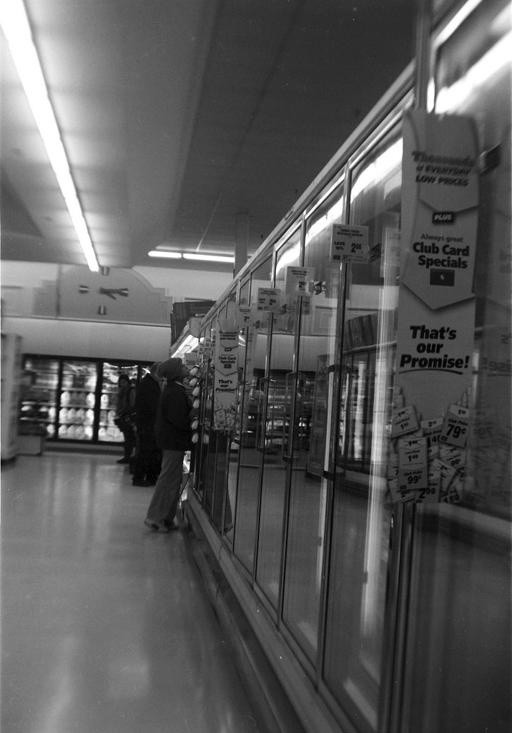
[115,358,193,532]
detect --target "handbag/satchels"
[114,414,135,432]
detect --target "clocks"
[77,266,129,315]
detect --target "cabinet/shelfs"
[17,354,139,447]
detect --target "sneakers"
[145,516,177,532]
[133,478,157,486]
[117,456,130,464]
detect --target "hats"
[119,375,129,382]
[157,358,182,381]
